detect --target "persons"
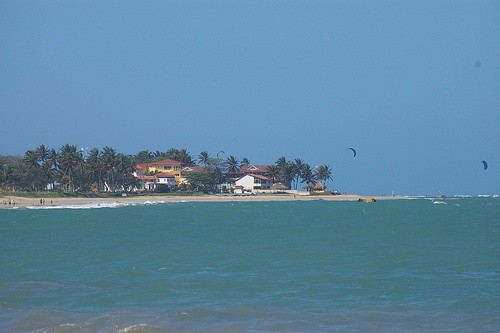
[97,202,101,206]
[12,200,15,207]
[39,199,42,206]
[51,199,53,205]
[2,199,7,207]
[42,198,45,206]
[8,199,12,206]
